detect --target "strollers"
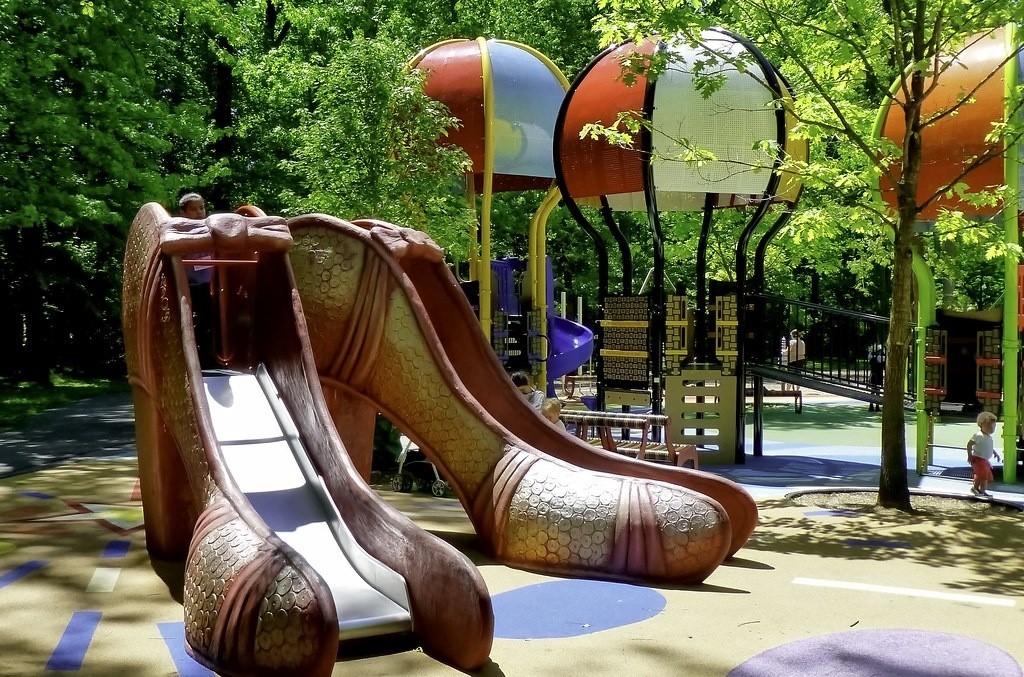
[391,439,452,497]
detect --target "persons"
[179,194,217,372]
[780,329,1000,497]
[512,372,566,433]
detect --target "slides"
[200,360,416,645]
[547,314,594,399]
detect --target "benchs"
[713,378,803,416]
[558,409,699,470]
